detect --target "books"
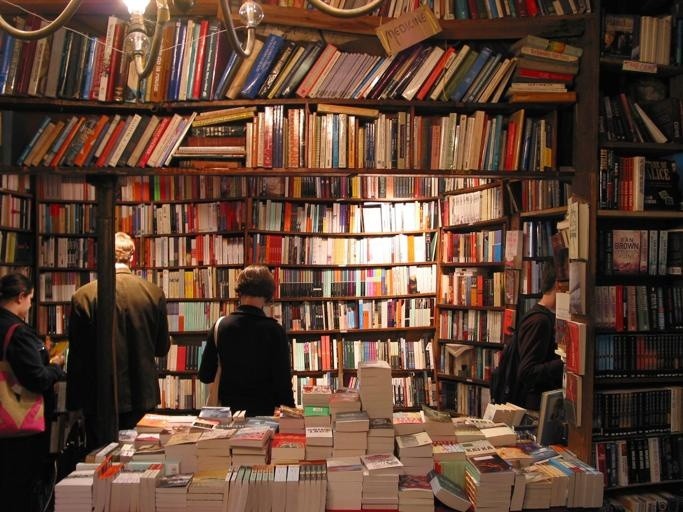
[1,0,590,173]
[438,178,525,417]
[1,173,98,454]
[520,181,568,313]
[556,13,682,511]
[113,176,443,410]
[56,361,604,511]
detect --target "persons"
[65,233,170,456]
[199,266,295,418]
[501,249,569,415]
[1,272,67,511]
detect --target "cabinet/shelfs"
[0,0,683,500]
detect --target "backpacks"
[490,307,552,404]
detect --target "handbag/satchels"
[0,360,48,439]
[204,363,222,408]
[60,416,89,478]
